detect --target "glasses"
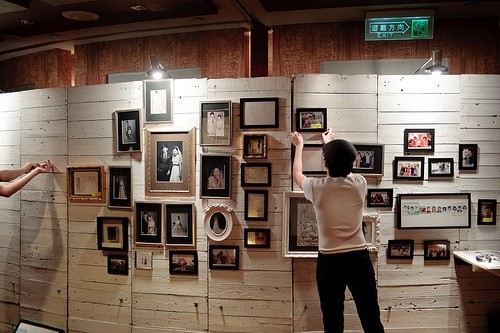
[321,148,327,160]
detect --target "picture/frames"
[15,319,65,333]
[64,81,496,277]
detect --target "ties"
[164,153,167,163]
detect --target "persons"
[402,205,467,214]
[172,216,185,235]
[0,160,53,197]
[208,167,224,189]
[214,251,232,264]
[409,136,431,147]
[371,193,389,204]
[206,112,224,136]
[114,176,127,198]
[141,212,155,234]
[396,244,409,256]
[157,144,182,182]
[484,205,492,219]
[178,257,193,270]
[430,248,446,257]
[399,164,418,177]
[248,139,263,154]
[466,150,473,165]
[301,113,319,128]
[124,121,133,142]
[355,152,374,169]
[290,128,385,333]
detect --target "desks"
[453,250,500,276]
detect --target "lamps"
[145,54,172,79]
[415,48,448,75]
